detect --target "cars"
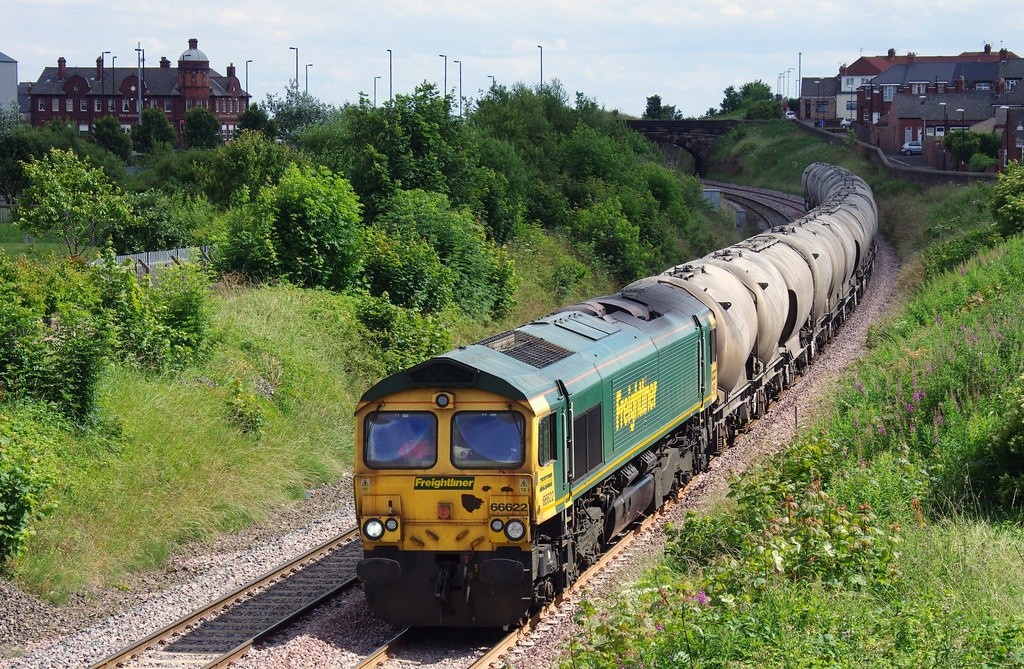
[840,117,857,129]
[785,111,797,120]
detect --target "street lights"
[135,47,145,112]
[956,108,965,132]
[113,56,116,118]
[488,76,494,99]
[306,64,312,95]
[374,77,381,107]
[939,103,947,138]
[246,60,252,109]
[538,46,542,93]
[814,80,820,109]
[848,86,852,130]
[387,50,392,105]
[102,52,111,96]
[289,47,298,90]
[454,61,461,117]
[439,55,446,99]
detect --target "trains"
[352,161,878,630]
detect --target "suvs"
[901,141,922,156]
